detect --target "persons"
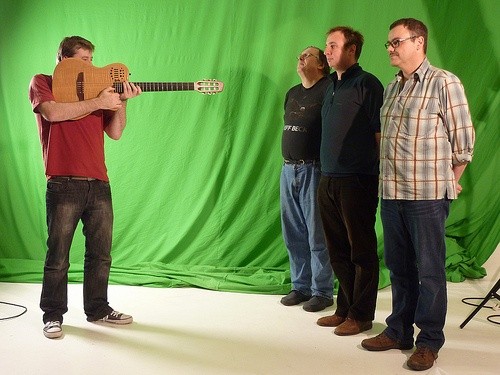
[361,18,476,371]
[281,46,334,313]
[317,27,384,336]
[29,36,142,337]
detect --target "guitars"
[51,57,224,120]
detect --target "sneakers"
[303,296,334,312]
[43,321,63,338]
[317,314,346,327]
[280,290,312,306]
[361,332,414,351]
[99,311,133,324]
[334,317,372,336]
[407,348,438,371]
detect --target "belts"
[56,176,96,181]
[284,159,313,165]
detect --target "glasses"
[296,52,320,61]
[384,36,417,50]
